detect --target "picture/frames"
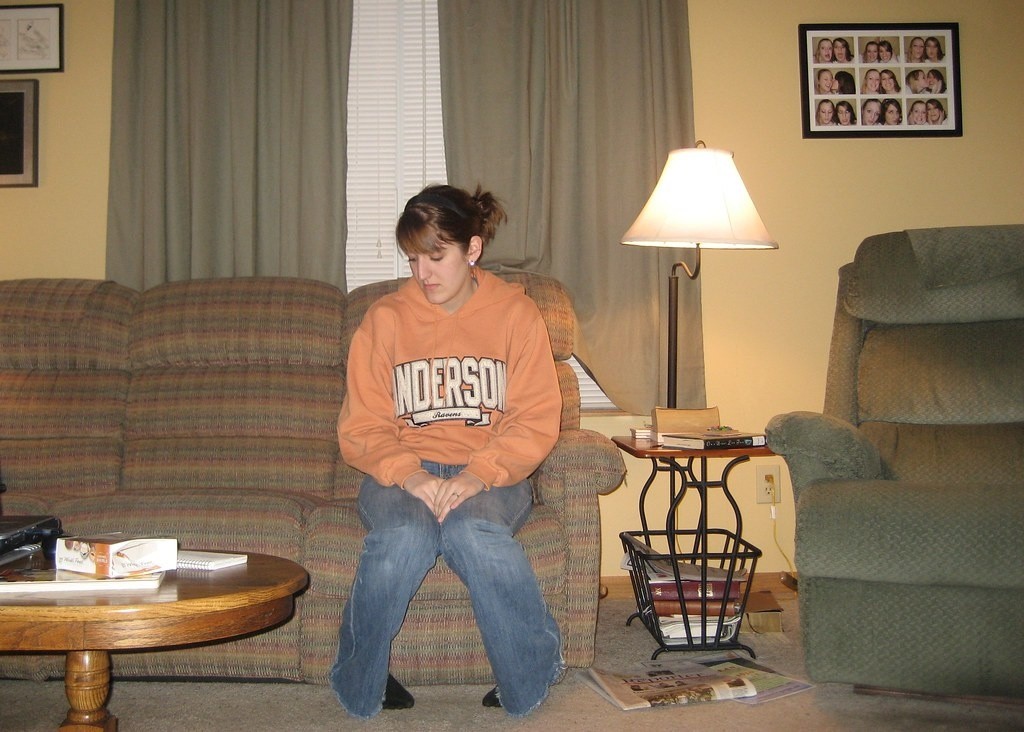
[0,79,39,188]
[0,3,64,73]
[798,22,963,139]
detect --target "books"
[630,405,784,646]
[0,550,248,591]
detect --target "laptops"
[0,515,62,554]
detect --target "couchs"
[765,224,1024,704]
[0,263,627,687]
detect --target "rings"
[452,492,460,498]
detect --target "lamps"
[620,148,779,409]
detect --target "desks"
[611,436,777,663]
[1,549,310,731]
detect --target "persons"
[328,184,569,718]
[813,36,948,126]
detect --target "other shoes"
[482,684,502,707]
[381,673,415,709]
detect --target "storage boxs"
[56,531,177,578]
